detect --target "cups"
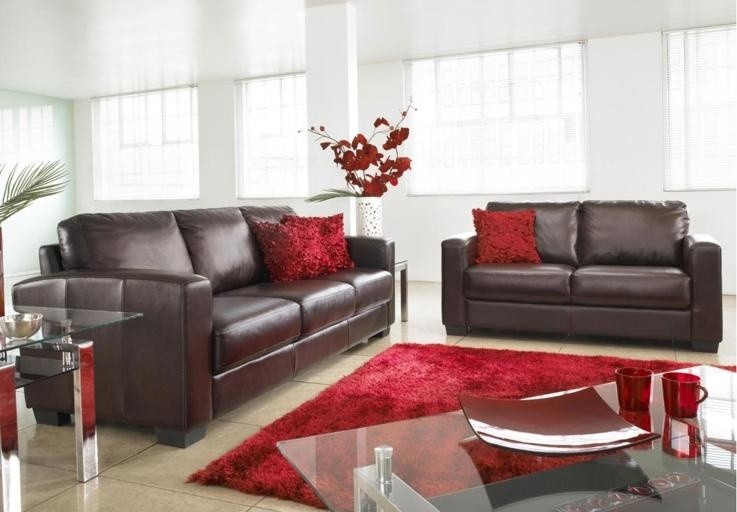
[615,366,653,408]
[663,372,707,419]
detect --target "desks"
[395,259,408,323]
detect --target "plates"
[459,384,660,456]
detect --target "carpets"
[184,343,737,511]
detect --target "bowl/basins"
[0,314,43,339]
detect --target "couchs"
[442,232,723,353]
[11,236,395,449]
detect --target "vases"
[356,197,382,239]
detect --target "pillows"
[58,205,355,293]
[471,202,689,265]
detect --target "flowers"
[298,94,417,203]
